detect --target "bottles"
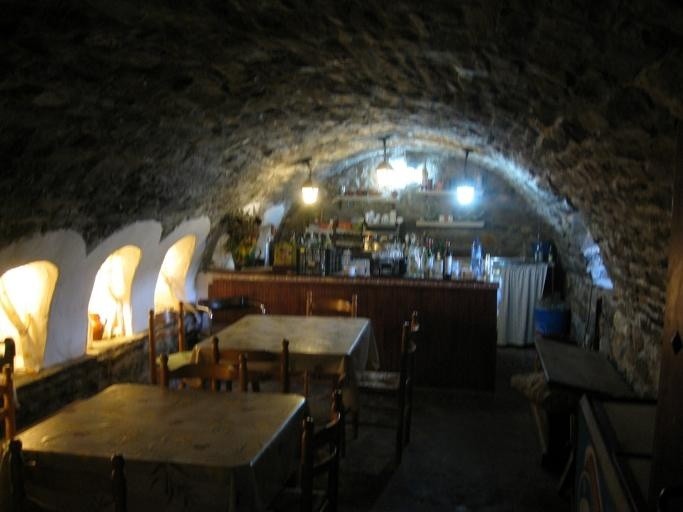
[420,159,429,190]
[87,313,104,340]
[520,233,554,263]
[298,232,337,276]
[470,237,493,281]
[403,230,453,281]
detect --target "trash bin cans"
[534,304,571,343]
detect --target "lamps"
[453,151,478,207]
[376,133,393,183]
[298,160,319,205]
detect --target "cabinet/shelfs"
[334,190,485,229]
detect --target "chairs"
[1,289,421,512]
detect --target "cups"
[343,266,356,278]
[365,209,404,227]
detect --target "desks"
[533,332,635,465]
